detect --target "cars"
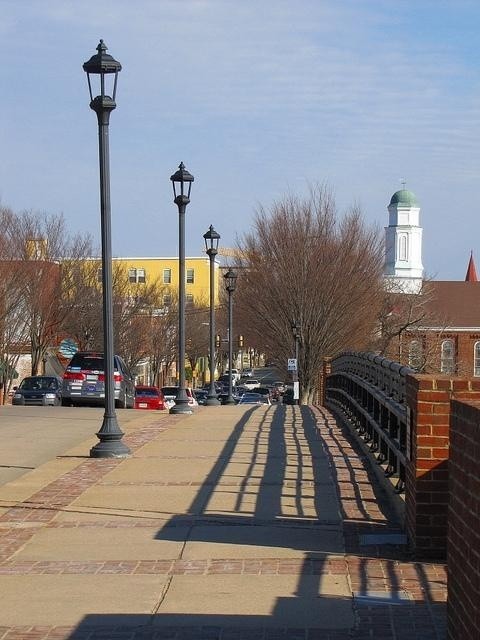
[12,350,289,410]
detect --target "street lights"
[223,267,237,405]
[203,224,221,406]
[82,39,131,457]
[169,162,195,414]
